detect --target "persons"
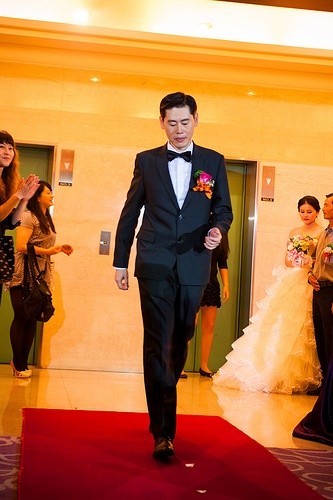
[306,191,333,402]
[177,216,230,378]
[113,91,234,459]
[208,195,327,396]
[0,130,41,316]
[3,178,74,380]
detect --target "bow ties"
[166,149,192,163]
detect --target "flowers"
[321,244,333,266]
[193,170,216,199]
[286,236,318,268]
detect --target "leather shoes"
[153,437,175,459]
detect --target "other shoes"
[180,371,187,378]
[198,367,214,377]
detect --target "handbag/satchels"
[21,243,57,323]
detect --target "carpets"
[0,436,333,500]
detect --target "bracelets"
[14,193,22,201]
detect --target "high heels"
[10,359,32,377]
